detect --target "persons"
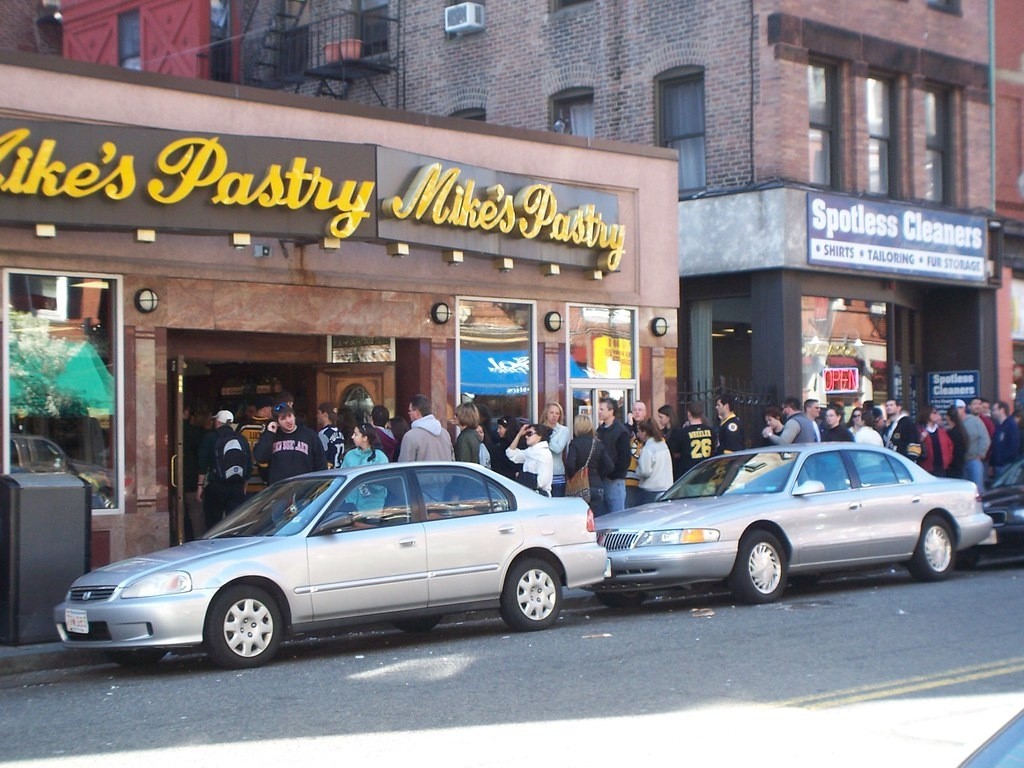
[506,420,555,499]
[174,403,252,546]
[397,395,457,511]
[241,386,1024,531]
[596,397,631,514]
[636,415,674,504]
[314,403,345,472]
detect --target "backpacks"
[213,429,246,482]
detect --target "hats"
[212,410,234,424]
[949,398,966,408]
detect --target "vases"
[324,39,363,63]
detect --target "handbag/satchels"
[564,465,590,503]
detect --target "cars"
[48,460,610,671]
[979,461,1024,548]
[588,442,998,603]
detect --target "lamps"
[134,288,159,313]
[543,264,560,277]
[496,258,513,272]
[136,229,156,242]
[232,233,251,249]
[431,303,452,324]
[319,236,341,253]
[586,269,603,279]
[443,251,463,267]
[544,311,562,332]
[34,224,56,237]
[651,317,668,337]
[387,242,409,258]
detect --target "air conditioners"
[444,2,486,34]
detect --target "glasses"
[527,431,538,437]
[852,414,861,418]
[274,401,287,411]
